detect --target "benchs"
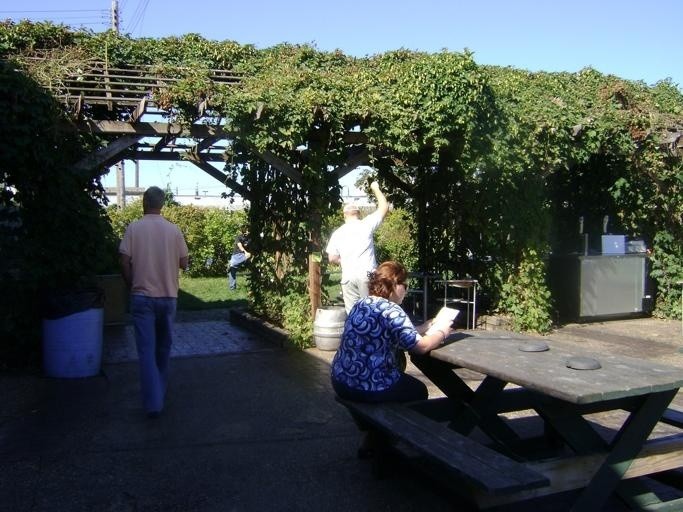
[335,391,550,511]
[615,404,683,431]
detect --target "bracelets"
[437,329,446,341]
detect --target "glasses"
[400,282,408,291]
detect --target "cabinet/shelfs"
[432,277,479,330]
[404,272,440,323]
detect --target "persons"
[325,182,388,317]
[226,224,254,290]
[119,186,190,420]
[330,259,453,481]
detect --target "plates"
[566,357,600,370]
[518,342,548,351]
